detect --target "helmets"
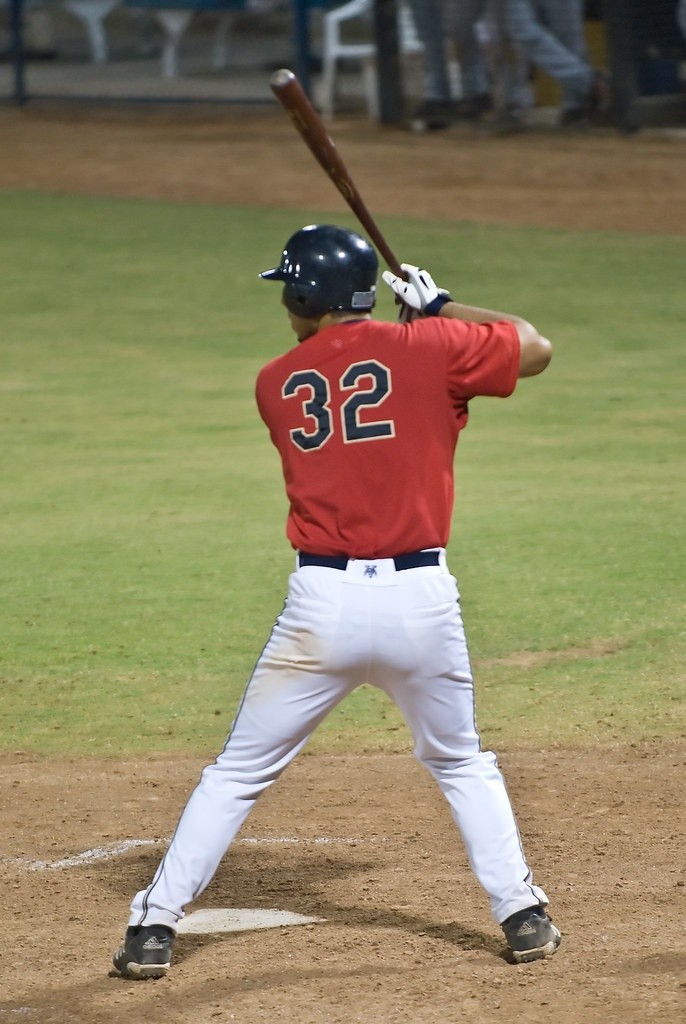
[259,224,378,319]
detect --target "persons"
[113,224,562,979]
[404,0,617,131]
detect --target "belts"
[299,552,440,571]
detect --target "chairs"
[316,1,465,123]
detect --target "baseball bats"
[270,67,404,278]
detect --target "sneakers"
[112,925,177,979]
[501,907,560,963]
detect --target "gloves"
[381,263,454,316]
